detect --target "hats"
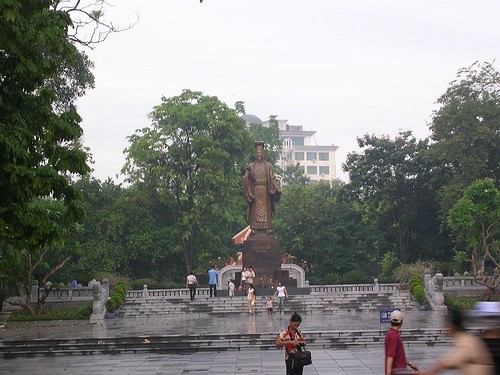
[464,301,500,316]
[390,310,404,323]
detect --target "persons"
[243,141,281,237]
[266,296,273,315]
[259,274,265,289]
[269,276,274,288]
[228,277,236,301]
[272,281,289,315]
[240,266,256,295]
[224,251,242,266]
[300,258,309,284]
[208,265,221,297]
[186,271,198,301]
[385,309,418,374]
[280,251,295,265]
[247,283,255,315]
[411,302,500,375]
[39,278,82,297]
[276,311,307,375]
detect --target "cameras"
[299,342,304,346]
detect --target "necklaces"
[290,327,295,333]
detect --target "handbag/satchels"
[294,344,312,366]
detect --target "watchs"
[409,361,414,365]
